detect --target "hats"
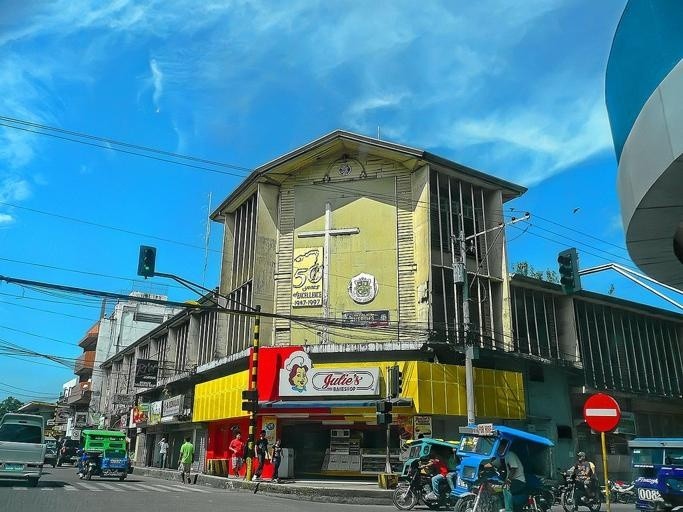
[577,452,586,456]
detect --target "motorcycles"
[599,477,637,504]
[76,429,132,481]
[392,422,556,512]
[558,468,601,512]
[626,436,683,512]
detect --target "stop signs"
[582,392,622,434]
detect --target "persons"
[77,447,99,472]
[177,437,195,484]
[564,451,600,503]
[229,430,285,483]
[157,438,169,470]
[420,454,448,501]
[499,442,527,512]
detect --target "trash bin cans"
[278,448,295,478]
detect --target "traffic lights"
[137,245,157,277]
[241,390,259,413]
[391,365,403,397]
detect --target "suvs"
[56,439,80,466]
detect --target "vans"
[0,412,47,487]
